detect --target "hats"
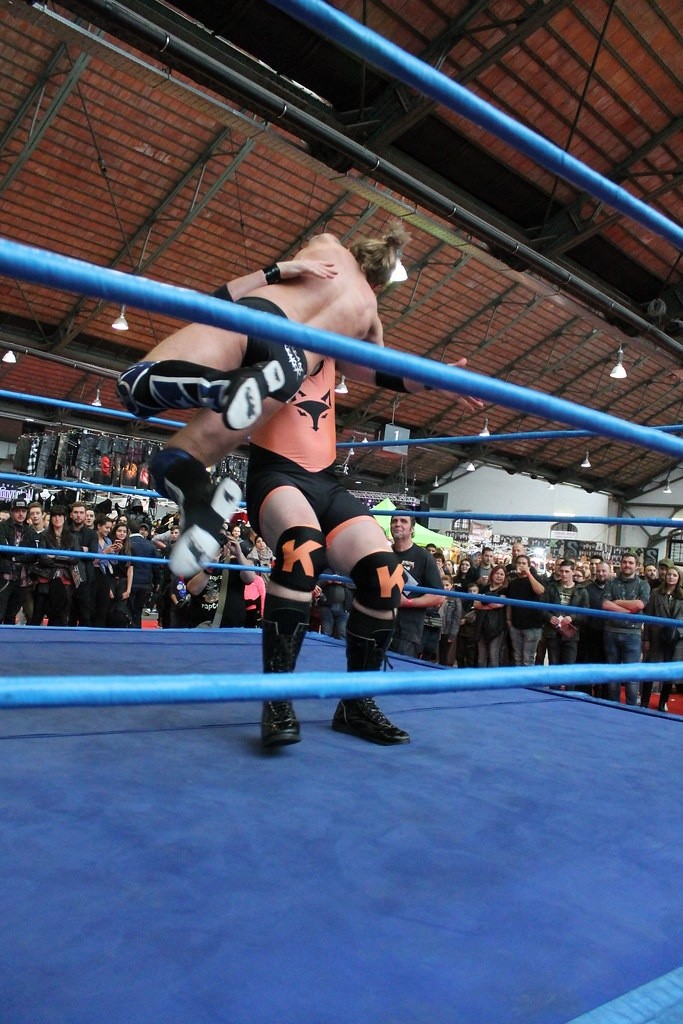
[141,523,149,530]
[11,499,28,510]
[659,559,674,568]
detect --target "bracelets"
[407,599,412,608]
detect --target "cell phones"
[114,540,122,550]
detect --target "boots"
[261,618,309,745]
[148,458,241,576]
[145,360,286,430]
[332,628,410,743]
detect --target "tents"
[371,498,453,559]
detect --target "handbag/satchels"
[465,610,476,623]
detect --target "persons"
[601,552,650,706]
[120,223,407,576]
[207,259,484,747]
[127,520,161,629]
[1,499,683,713]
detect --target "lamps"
[610,340,628,378]
[433,476,439,487]
[362,432,368,442]
[389,250,408,281]
[334,374,349,393]
[663,480,673,494]
[466,460,476,471]
[91,389,102,406]
[580,452,591,467]
[349,448,355,455]
[2,351,16,363]
[479,419,490,436]
[112,304,129,330]
[405,482,409,491]
